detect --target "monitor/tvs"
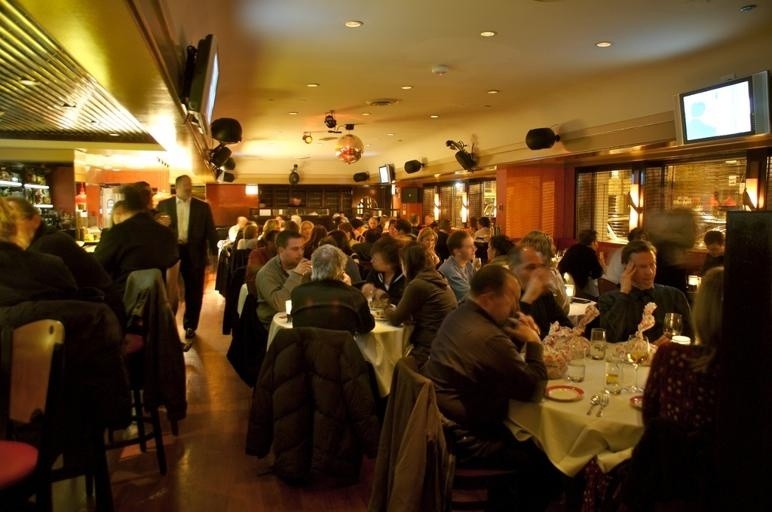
[379,165,392,185]
[186,30,222,136]
[673,71,770,146]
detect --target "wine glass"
[625,335,650,393]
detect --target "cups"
[375,309,385,318]
[567,350,586,383]
[605,359,621,391]
[590,328,606,360]
[688,274,701,286]
[663,312,682,338]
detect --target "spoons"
[586,392,600,416]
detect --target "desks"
[567,296,598,330]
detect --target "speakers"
[455,152,474,170]
[226,159,235,169]
[211,118,242,143]
[353,172,368,181]
[405,161,420,174]
[401,187,420,203]
[526,129,556,150]
[224,173,234,183]
[212,147,231,167]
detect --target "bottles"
[0,166,65,230]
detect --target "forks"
[595,395,610,417]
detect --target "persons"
[2,175,725,511]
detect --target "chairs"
[1,293,132,512]
[245,327,380,492]
[653,266,687,300]
[368,356,517,510]
[0,319,65,510]
[104,268,186,475]
[220,249,265,388]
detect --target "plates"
[630,397,644,410]
[544,385,585,401]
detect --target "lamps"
[335,124,364,165]
